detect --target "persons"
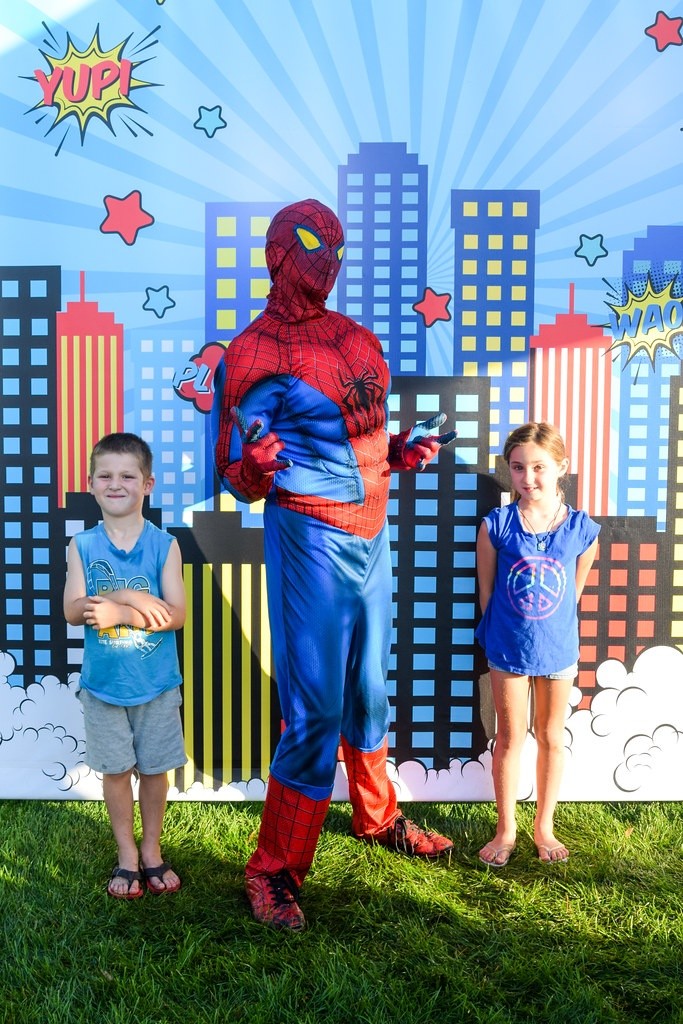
[213,200,458,932]
[474,421,602,868]
[64,432,186,898]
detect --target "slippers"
[539,841,570,865]
[107,858,144,900]
[478,840,517,867]
[140,858,181,895]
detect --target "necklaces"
[519,502,562,551]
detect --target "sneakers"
[372,812,455,859]
[243,870,305,932]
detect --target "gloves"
[403,414,458,474]
[231,408,293,481]
